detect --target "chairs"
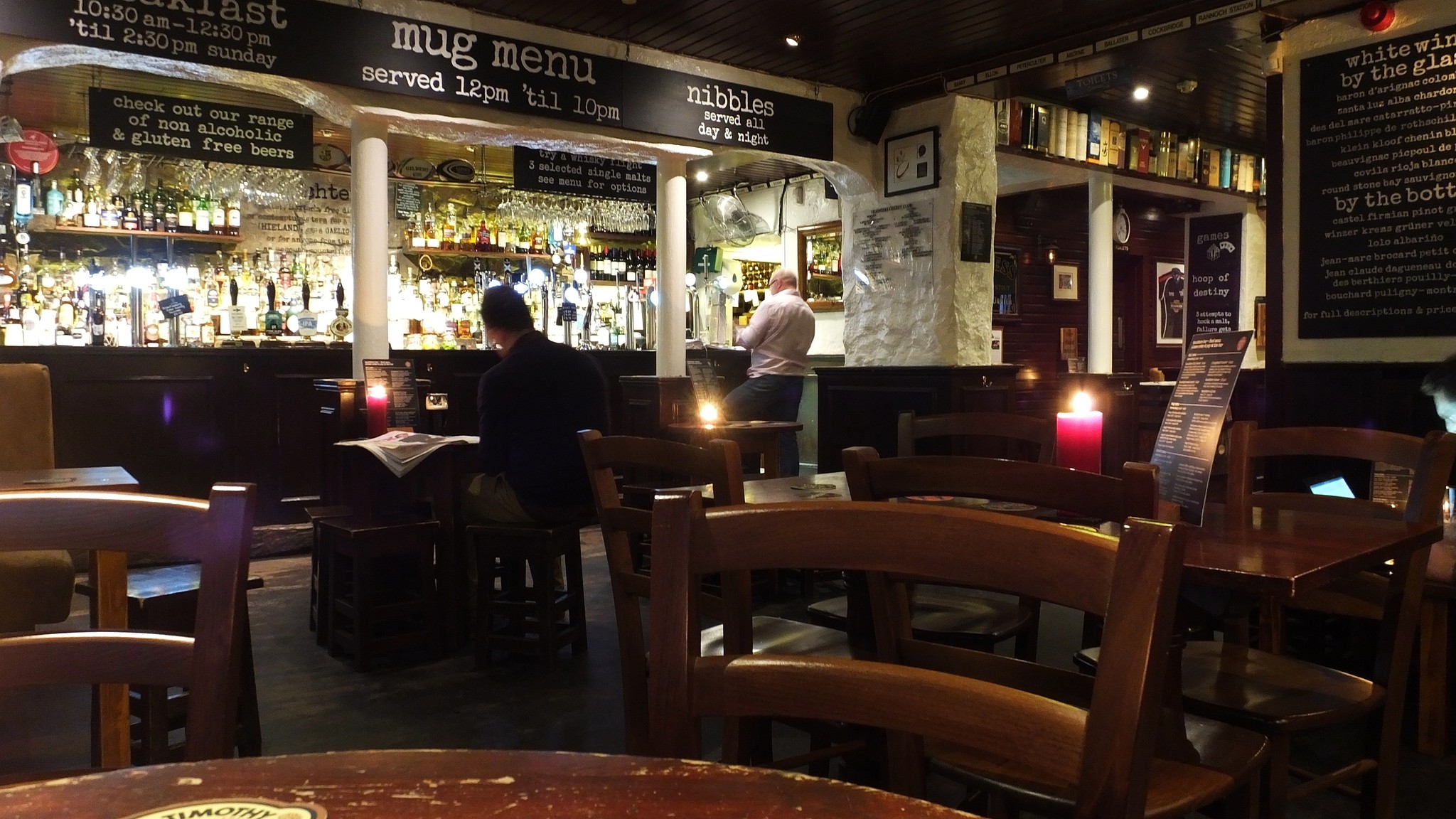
[1083,414,1456,819]
[846,404,1073,666]
[0,476,264,790]
[0,363,74,639]
[838,442,1275,818]
[641,493,1175,818]
[568,425,889,788]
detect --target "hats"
[482,285,526,326]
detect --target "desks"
[623,455,1450,819]
[666,419,806,486]
[331,432,482,666]
[0,744,982,819]
[1,463,145,769]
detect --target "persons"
[1421,349,1456,583]
[722,268,816,478]
[458,282,609,622]
[395,432,446,444]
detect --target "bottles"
[12,160,242,239]
[389,254,627,348]
[589,243,657,282]
[808,248,843,277]
[998,99,1010,145]
[0,240,351,348]
[403,202,588,255]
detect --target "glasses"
[767,279,776,288]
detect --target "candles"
[366,384,389,438]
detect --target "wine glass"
[495,188,657,233]
[81,144,312,210]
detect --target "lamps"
[784,31,802,47]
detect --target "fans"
[704,192,764,248]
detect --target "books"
[332,428,481,479]
[993,97,1266,197]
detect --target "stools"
[303,502,398,651]
[456,515,590,666]
[73,560,265,769]
[320,517,447,678]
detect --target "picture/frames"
[884,124,939,198]
